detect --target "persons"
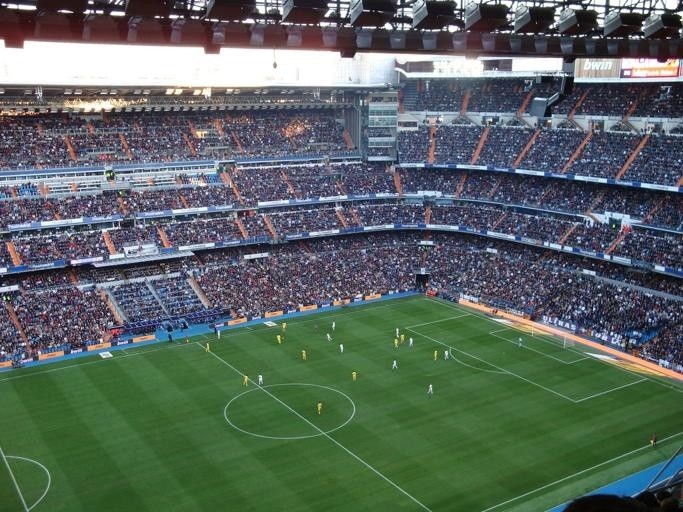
[242,374,248,387]
[560,482,683,511]
[0,76,385,369]
[351,370,356,382]
[652,432,656,446]
[257,372,263,386]
[427,384,433,394]
[317,401,321,415]
[386,80,682,373]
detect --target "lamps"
[35,0,683,58]
